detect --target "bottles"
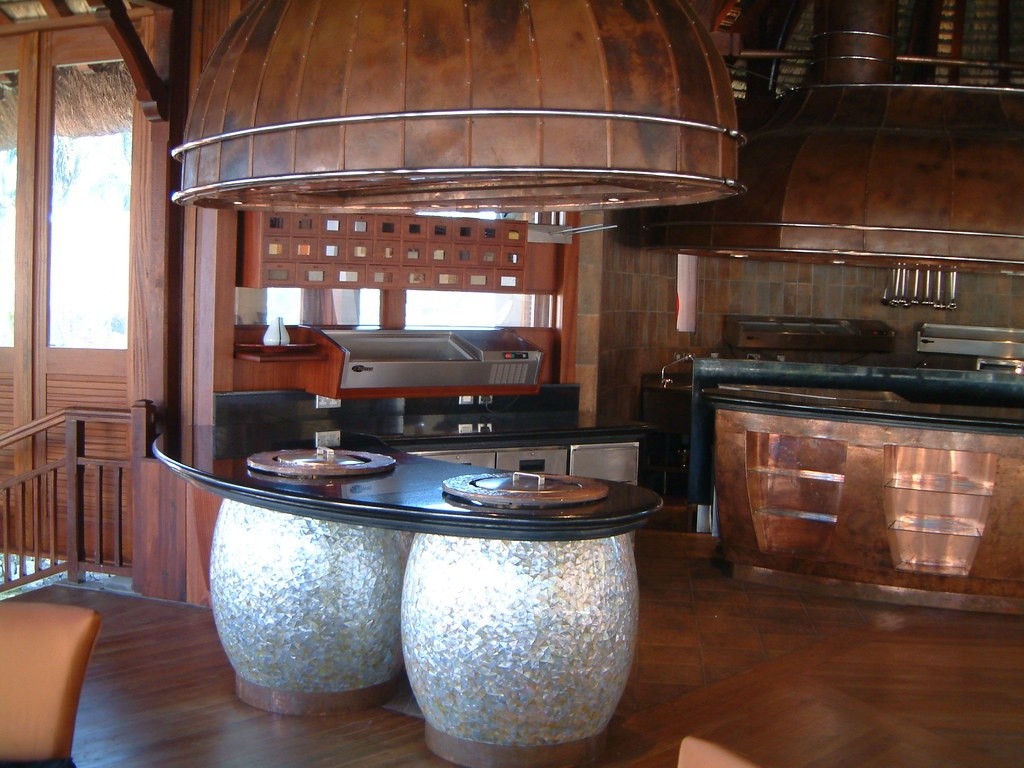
[263,316,290,345]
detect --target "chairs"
[0,595,101,768]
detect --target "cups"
[696,505,712,533]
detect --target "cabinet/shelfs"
[748,464,843,525]
[883,474,991,539]
